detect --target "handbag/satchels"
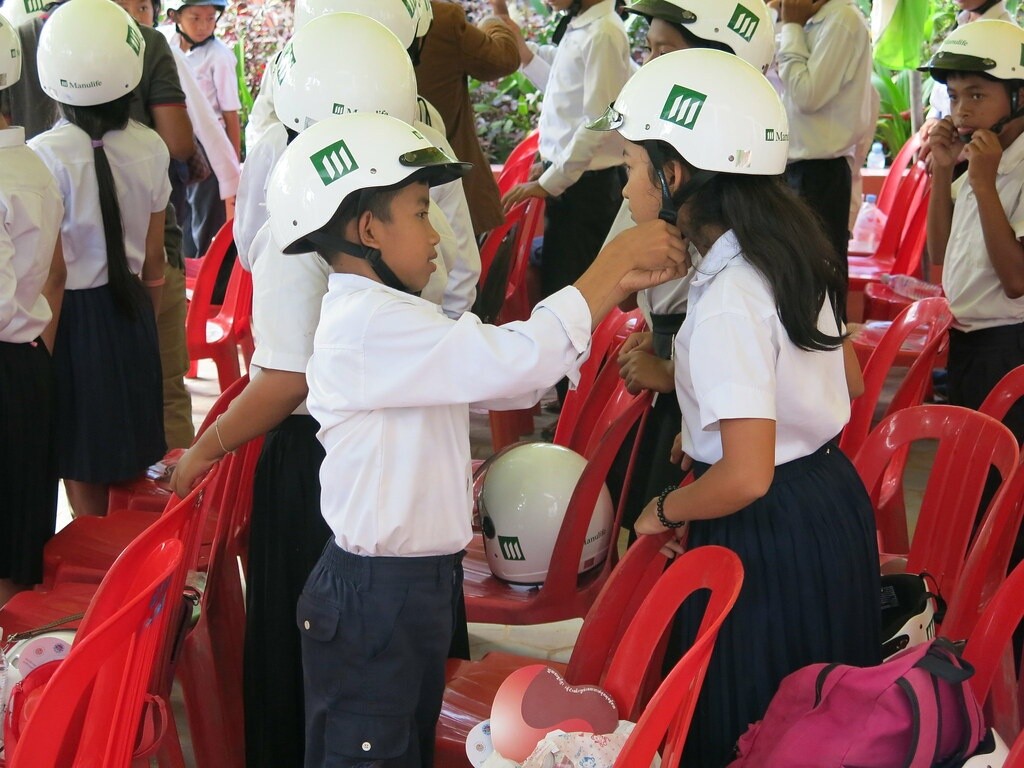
[166,586,200,698]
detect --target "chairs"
[0,133,1024,768]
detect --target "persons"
[169,1,482,768]
[0,0,243,605]
[530,0,882,528]
[266,113,686,768]
[918,1,1024,684]
[583,48,881,768]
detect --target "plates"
[465,717,494,768]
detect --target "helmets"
[644,0,776,76]
[613,48,789,175]
[164,0,232,18]
[267,113,474,254]
[880,573,946,663]
[37,0,145,106]
[0,14,21,91]
[917,19,1024,84]
[273,12,417,134]
[478,443,613,585]
[293,0,433,50]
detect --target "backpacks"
[726,636,996,768]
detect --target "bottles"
[880,272,942,301]
[866,142,886,170]
[852,194,876,251]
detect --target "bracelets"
[216,414,231,453]
[657,486,684,528]
